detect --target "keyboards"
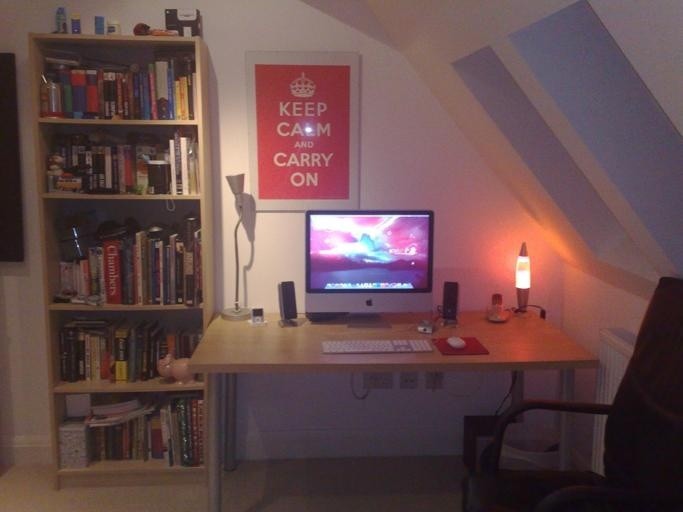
[323,338,433,354]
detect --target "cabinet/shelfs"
[28,32,214,491]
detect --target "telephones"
[489,293,510,322]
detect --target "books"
[59,322,203,382]
[60,220,202,305]
[46,56,193,120]
[49,126,199,195]
[87,393,205,468]
[162,6,201,36]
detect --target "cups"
[147,159,171,194]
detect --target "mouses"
[447,335,466,348]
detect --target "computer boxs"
[462,415,500,482]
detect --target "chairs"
[475,278,683,512]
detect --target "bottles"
[156,354,196,385]
[107,20,122,36]
[94,16,104,34]
[54,6,66,33]
[491,294,503,318]
[39,79,63,119]
[70,13,82,34]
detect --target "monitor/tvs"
[305,208,438,330]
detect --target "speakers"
[277,281,298,327]
[440,281,458,327]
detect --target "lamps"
[515,242,532,312]
[221,172,251,322]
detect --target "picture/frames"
[245,50,361,212]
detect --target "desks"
[190,308,600,512]
